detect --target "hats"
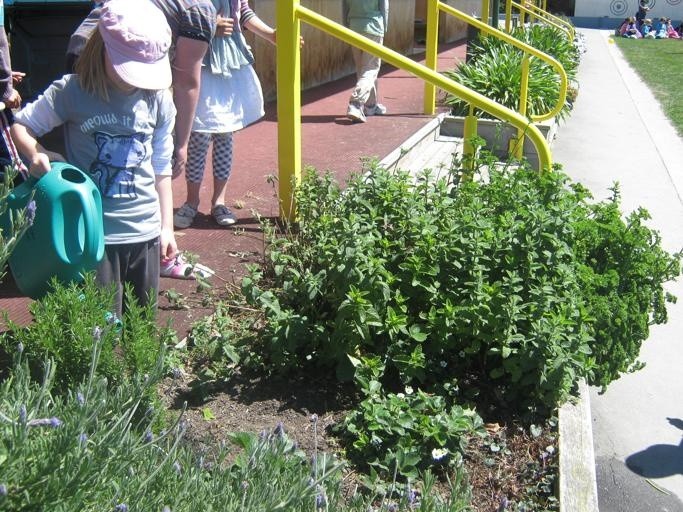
[97,0,172,91]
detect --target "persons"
[69,0,305,278]
[0,1,32,187]
[340,0,388,121]
[616,1,682,41]
[12,1,180,327]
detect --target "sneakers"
[211,205,238,226]
[173,203,197,228]
[160,250,214,280]
[363,104,387,116]
[347,104,366,123]
